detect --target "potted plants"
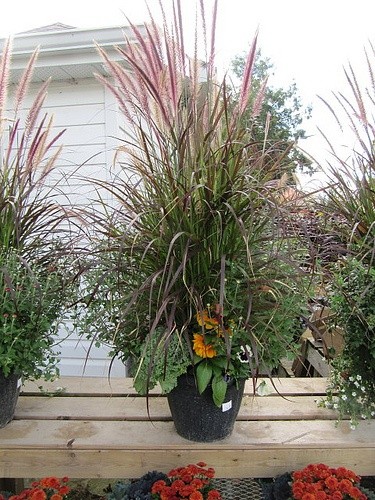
[1,35,84,430]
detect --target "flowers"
[0,461,218,500]
[291,463,367,500]
[76,0,305,429]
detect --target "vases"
[159,373,246,440]
